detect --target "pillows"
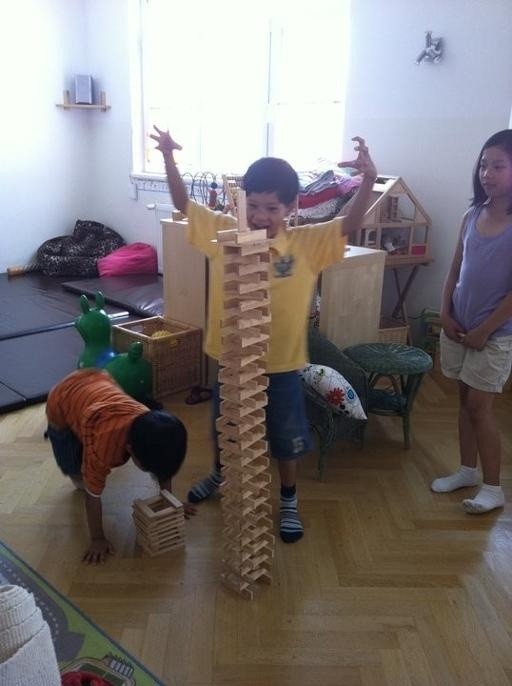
[303,362,368,422]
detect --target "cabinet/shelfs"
[158,216,389,404]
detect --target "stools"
[344,343,434,451]
[98,243,158,278]
[38,220,123,277]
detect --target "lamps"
[414,31,445,66]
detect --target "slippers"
[185,385,212,405]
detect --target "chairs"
[302,324,380,486]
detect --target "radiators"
[154,204,177,275]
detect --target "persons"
[45,365,200,570]
[429,127,512,516]
[146,124,377,547]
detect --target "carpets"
[0,539,167,686]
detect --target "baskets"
[112,317,201,399]
[378,316,410,345]
